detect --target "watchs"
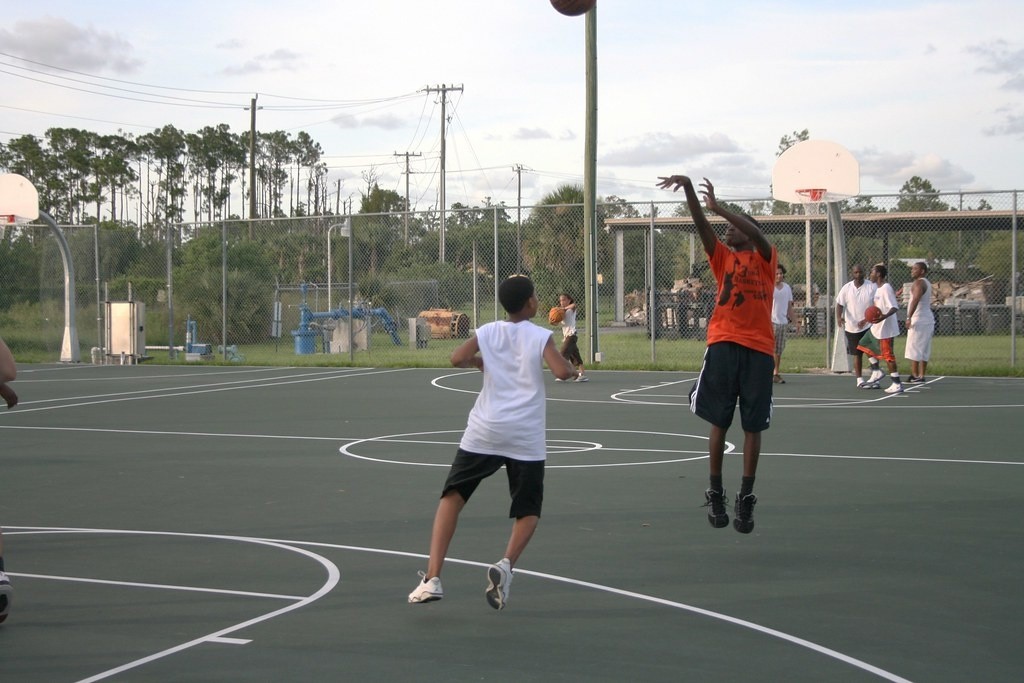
[906,317,910,320]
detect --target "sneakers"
[485,557,515,609]
[867,370,886,383]
[863,381,880,389]
[555,377,572,383]
[884,383,904,394]
[407,570,443,604]
[700,487,730,528]
[901,375,926,384]
[857,378,865,388]
[734,490,757,533]
[573,373,589,382]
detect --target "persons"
[656,174,779,534]
[771,264,801,384]
[408,276,580,612]
[901,262,935,385]
[835,264,877,390]
[549,293,589,383]
[858,264,905,394]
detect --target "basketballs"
[864,307,884,325]
[548,307,565,323]
[549,0,597,18]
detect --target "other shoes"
[0,573,12,623]
[773,374,785,384]
